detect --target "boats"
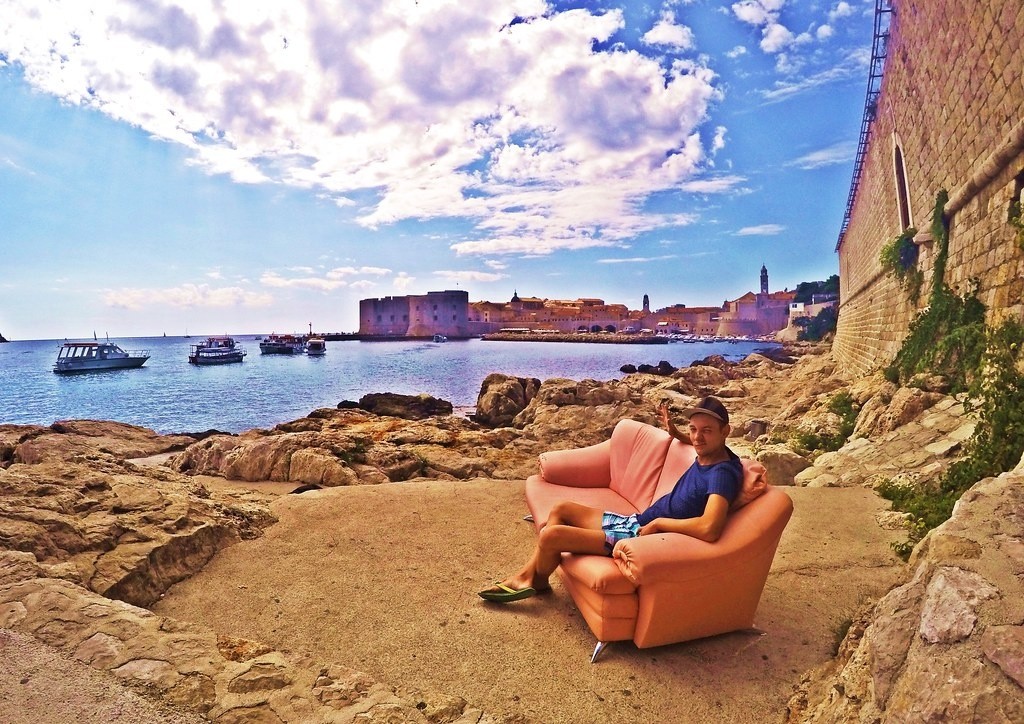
[657,333,762,344]
[188,337,249,366]
[306,338,327,355]
[432,334,449,344]
[259,333,306,354]
[51,341,153,374]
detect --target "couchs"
[522,418,793,663]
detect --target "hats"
[682,397,729,424]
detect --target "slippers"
[534,586,552,595]
[478,584,536,602]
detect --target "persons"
[478,397,744,603]
